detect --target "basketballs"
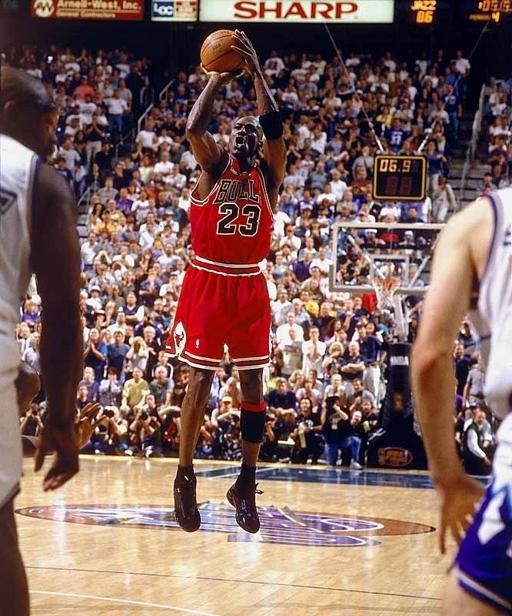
[201,29,245,74]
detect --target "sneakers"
[225,482,264,536]
[348,458,362,473]
[171,474,202,534]
[123,448,154,458]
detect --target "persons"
[405,187,512,615]
[2,60,88,615]
[160,28,289,535]
[1,36,512,477]
